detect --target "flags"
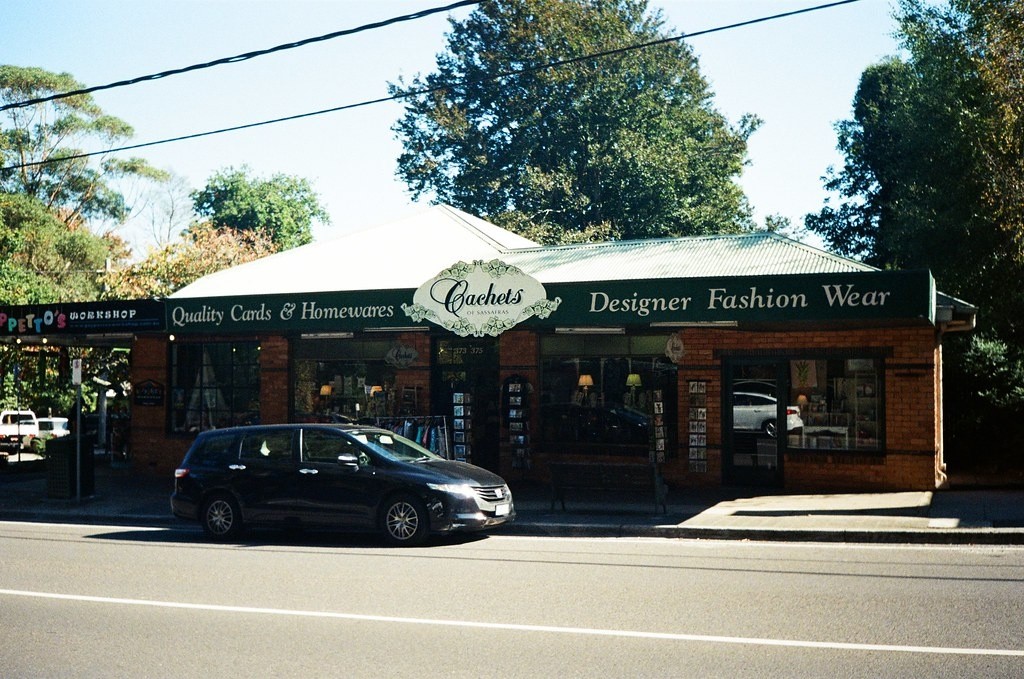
[11,362,21,395]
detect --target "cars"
[733,392,804,438]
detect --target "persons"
[66,402,88,433]
[73,395,90,412]
[113,399,127,414]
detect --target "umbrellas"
[187,349,227,427]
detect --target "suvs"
[170,424,516,546]
[0,409,118,454]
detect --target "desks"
[803,426,851,449]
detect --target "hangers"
[381,415,444,427]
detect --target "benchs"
[546,460,668,517]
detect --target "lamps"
[797,394,809,412]
[577,374,594,405]
[370,386,382,411]
[625,373,642,410]
[320,385,331,412]
